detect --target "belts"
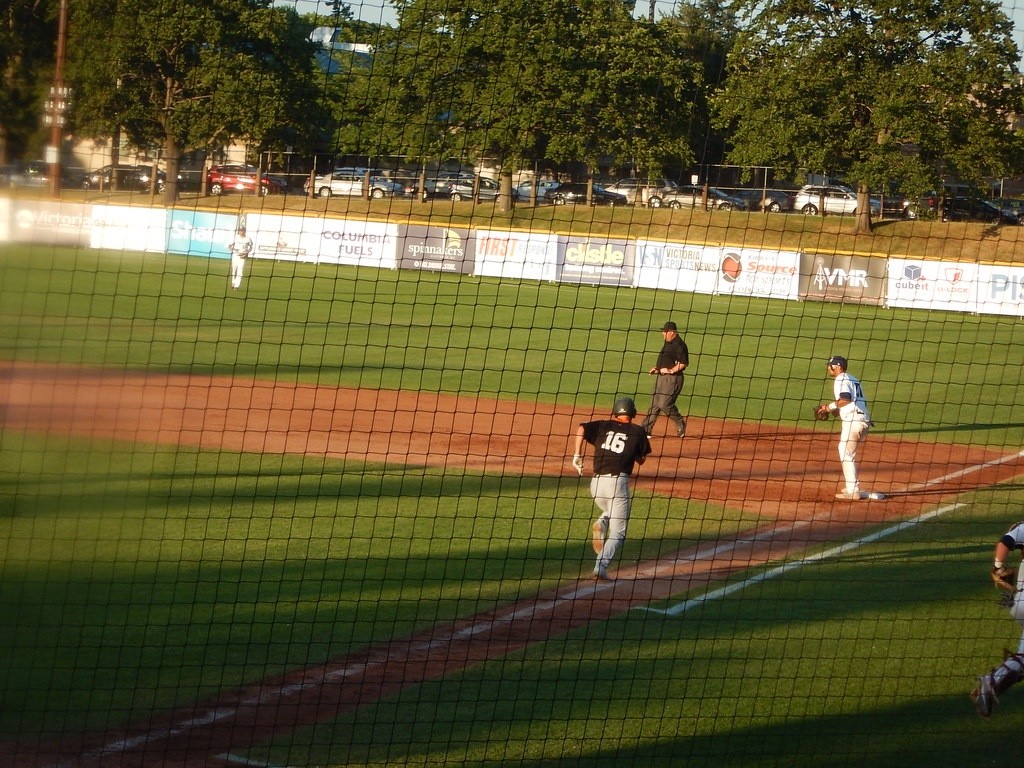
[611,472,618,476]
[857,412,864,414]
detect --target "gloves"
[573,455,584,476]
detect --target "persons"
[572,398,652,580]
[818,355,871,501]
[228,227,252,291]
[640,321,688,439]
[971,521,1024,718]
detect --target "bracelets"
[829,402,837,411]
[670,369,674,374]
[995,557,1004,568]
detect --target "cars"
[0,161,1024,225]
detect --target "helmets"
[613,397,636,418]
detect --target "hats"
[660,322,677,331]
[826,356,847,366]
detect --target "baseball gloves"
[813,405,830,421]
[992,566,1018,592]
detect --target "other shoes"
[646,434,652,438]
[594,566,608,580]
[835,491,861,500]
[677,422,685,438]
[841,488,848,493]
[592,518,607,554]
[976,675,1000,719]
